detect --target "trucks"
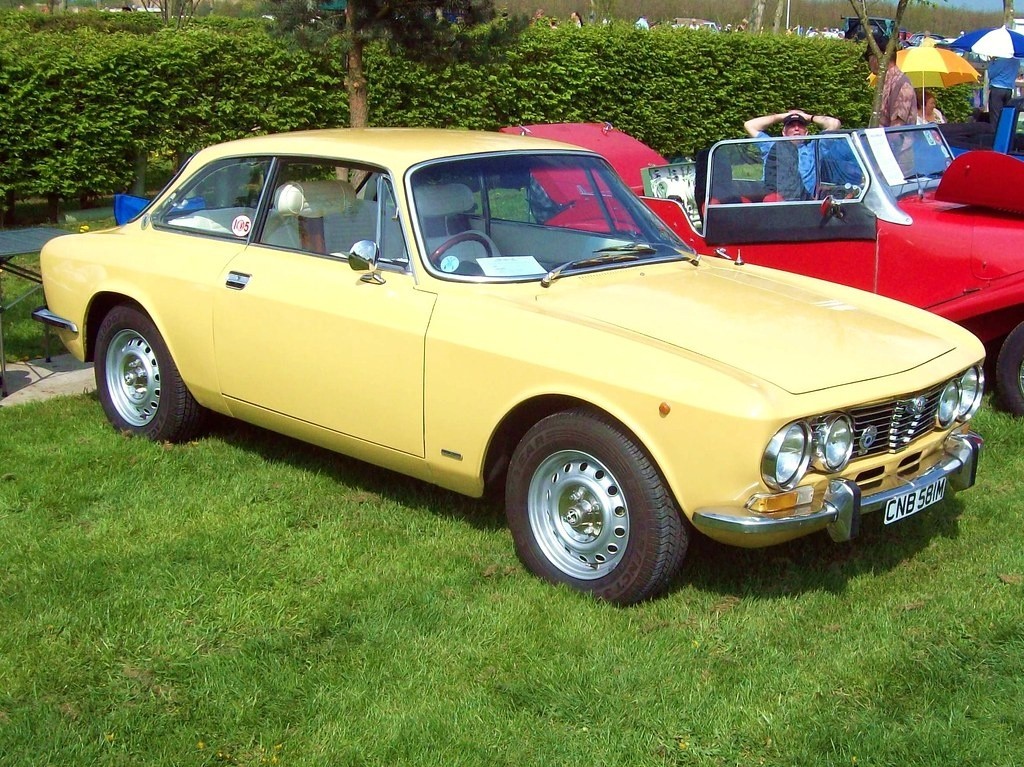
[845,17,895,43]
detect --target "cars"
[901,34,947,49]
[824,94,1024,178]
[499,118,1024,415]
[32,126,986,609]
[702,22,721,32]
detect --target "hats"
[784,114,807,126]
[864,36,897,56]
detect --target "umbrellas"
[867,47,982,124]
[949,25,1024,59]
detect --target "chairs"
[416,182,506,268]
[276,180,377,256]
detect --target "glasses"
[864,53,874,62]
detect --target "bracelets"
[811,115,815,123]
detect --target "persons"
[744,110,841,200]
[959,30,964,38]
[121,6,132,12]
[42,8,48,12]
[799,26,838,36]
[718,23,722,30]
[965,108,980,123]
[735,22,748,32]
[726,23,733,33]
[690,19,699,30]
[502,13,509,19]
[838,33,844,39]
[536,9,545,19]
[917,92,939,127]
[633,15,654,31]
[858,36,917,180]
[988,56,1020,126]
[572,11,583,28]
[18,4,24,9]
[550,22,557,28]
[919,31,936,47]
